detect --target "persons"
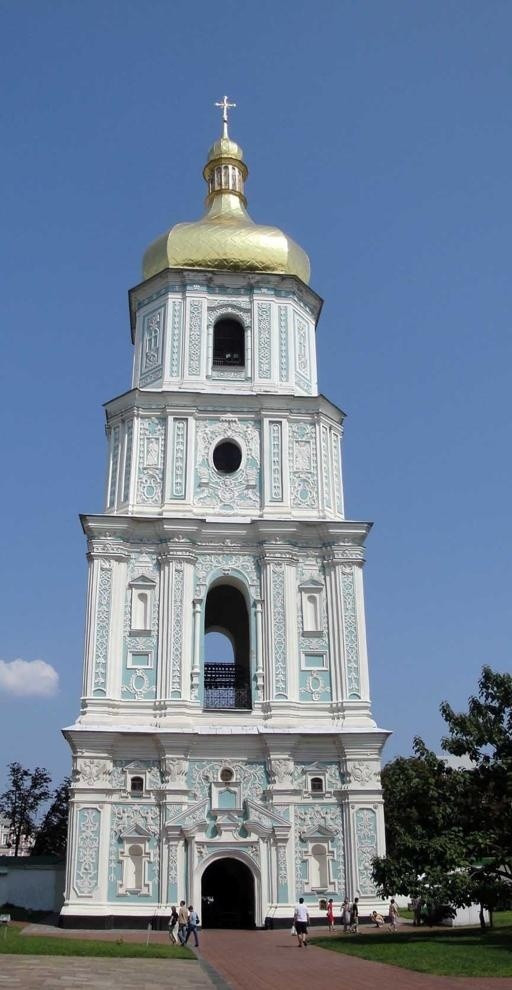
[348,897,360,933]
[326,898,337,932]
[411,895,426,926]
[181,904,200,947]
[340,897,352,932]
[168,906,179,945]
[387,899,400,932]
[370,910,385,928]
[292,897,311,948]
[178,901,188,945]
